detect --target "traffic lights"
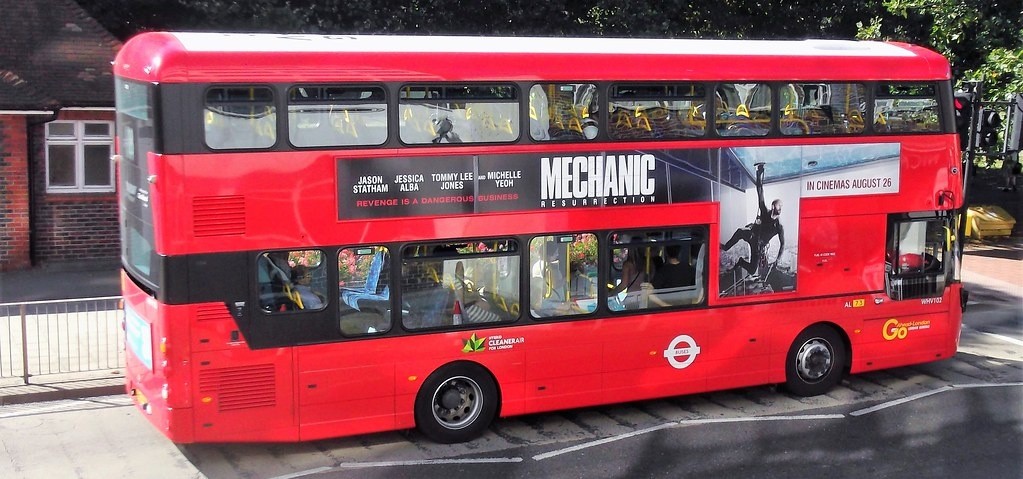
[977,108,1001,148]
[954,92,974,148]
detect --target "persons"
[719,162,785,275]
[650,246,694,289]
[291,265,323,309]
[607,236,647,297]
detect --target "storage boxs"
[967,206,1016,239]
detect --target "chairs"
[254,102,892,142]
[342,252,392,311]
[423,266,590,321]
[283,284,323,309]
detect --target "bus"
[110,31,970,446]
